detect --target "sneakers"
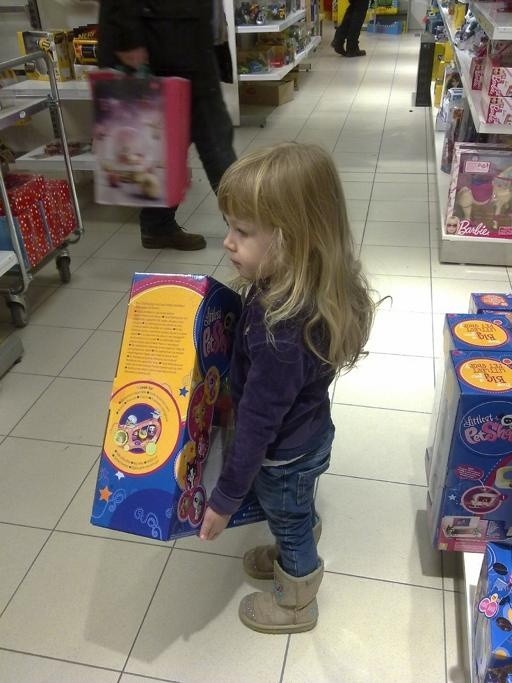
[347,49,366,57]
[141,226,208,251]
[330,37,345,55]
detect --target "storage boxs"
[89,271,270,540]
[424,291,512,683]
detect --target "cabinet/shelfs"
[1,75,202,173]
[224,0,322,82]
[420,0,512,268]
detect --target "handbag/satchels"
[88,67,191,210]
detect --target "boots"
[239,558,325,633]
[242,512,322,581]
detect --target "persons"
[195,142,393,633]
[331,0,369,57]
[95,0,238,251]
